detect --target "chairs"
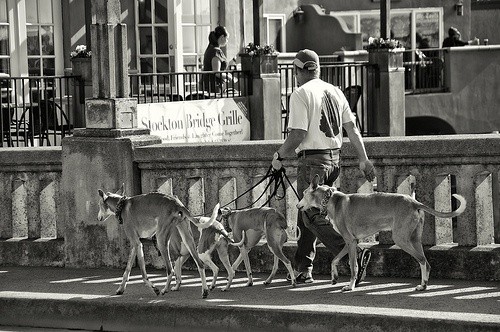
[1,100,73,146]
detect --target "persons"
[389,24,476,88]
[272,48,376,285]
[203,25,238,94]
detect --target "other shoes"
[287,270,313,284]
[356,249,371,285]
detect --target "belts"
[297,149,340,158]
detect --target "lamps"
[456,0,463,17]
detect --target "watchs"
[273,151,284,161]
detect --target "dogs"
[170,216,246,296]
[217,207,302,288]
[96,183,221,301]
[296,173,467,291]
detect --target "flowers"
[245,42,277,56]
[70,44,92,58]
[365,39,404,50]
[341,86,363,137]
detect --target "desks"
[0,103,39,147]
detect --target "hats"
[294,49,319,71]
